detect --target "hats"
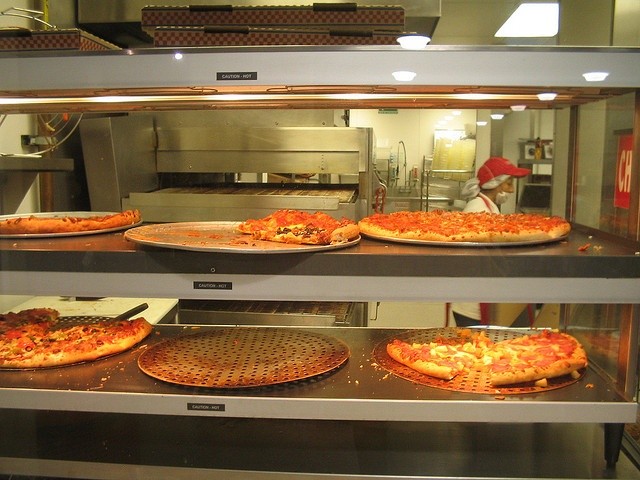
[477,157,532,187]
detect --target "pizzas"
[235,210,359,244]
[386,339,490,380]
[485,330,589,385]
[0,209,140,233]
[360,210,572,241]
[0,309,151,368]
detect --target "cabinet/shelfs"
[0,45,639,477]
[420,157,478,211]
[517,159,553,213]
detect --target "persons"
[451,159,532,328]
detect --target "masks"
[495,191,512,206]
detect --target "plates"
[360,214,572,246]
[0,211,145,239]
[124,221,361,253]
